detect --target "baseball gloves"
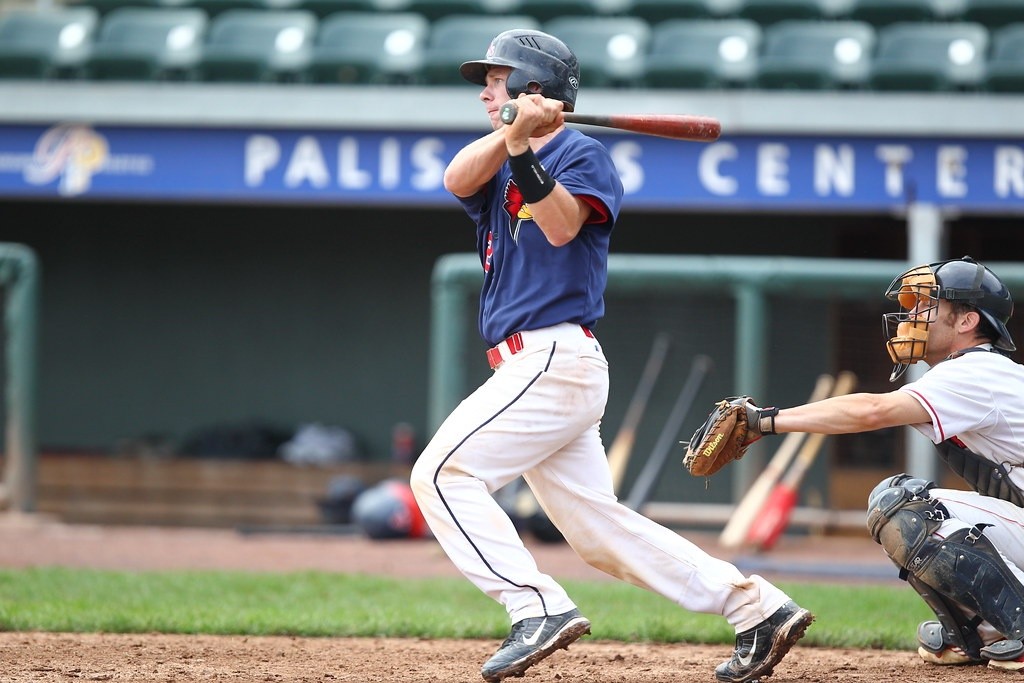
[682,395,780,477]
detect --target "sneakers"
[715,599,813,683]
[987,652,1024,670]
[481,608,591,683]
[918,635,1008,664]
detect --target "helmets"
[459,28,580,112]
[929,256,1017,352]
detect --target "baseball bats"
[717,374,836,549]
[747,371,860,553]
[502,102,721,143]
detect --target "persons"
[409,27,815,681]
[681,255,1023,670]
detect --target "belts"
[485,324,594,370]
